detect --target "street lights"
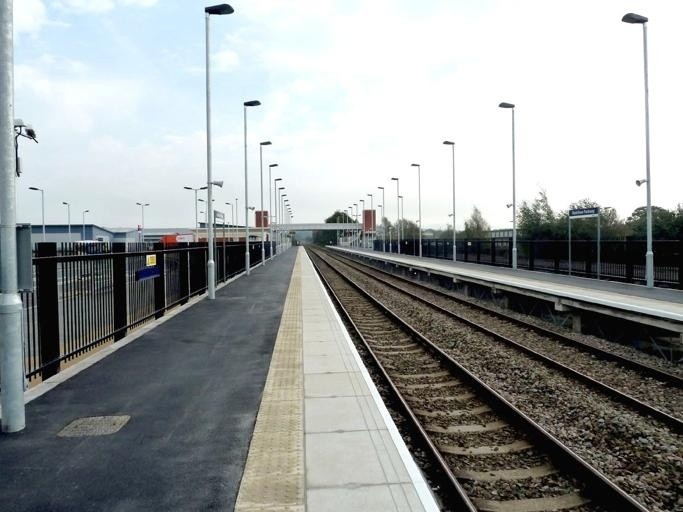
[183,187,208,241]
[201,4,237,300]
[242,101,262,276]
[622,13,657,287]
[412,163,423,259]
[82,210,89,241]
[63,203,71,242]
[136,203,150,242]
[31,188,46,242]
[268,163,294,257]
[499,102,520,269]
[339,177,404,256]
[259,142,273,266]
[443,142,457,262]
